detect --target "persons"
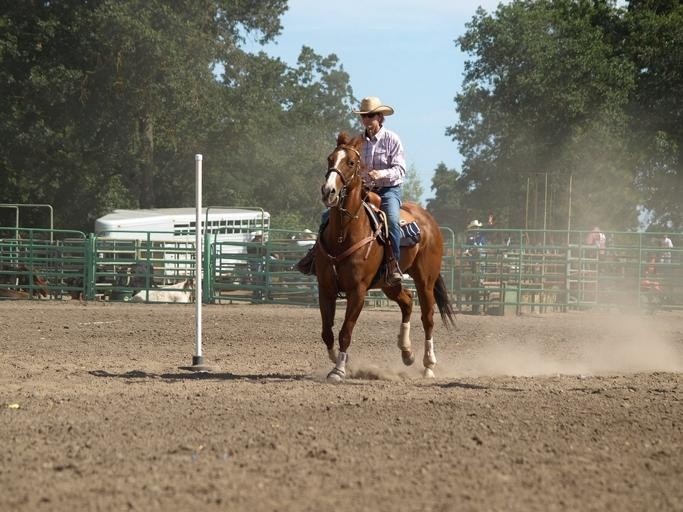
[464,215,494,276]
[292,95,404,287]
[659,234,674,264]
[247,230,277,304]
[290,228,318,303]
[588,227,606,271]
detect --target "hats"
[250,230,262,236]
[467,219,482,228]
[352,96,394,115]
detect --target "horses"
[313,130,457,386]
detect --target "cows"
[0,260,197,304]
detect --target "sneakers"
[381,272,401,288]
[298,253,314,273]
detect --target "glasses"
[360,113,378,118]
